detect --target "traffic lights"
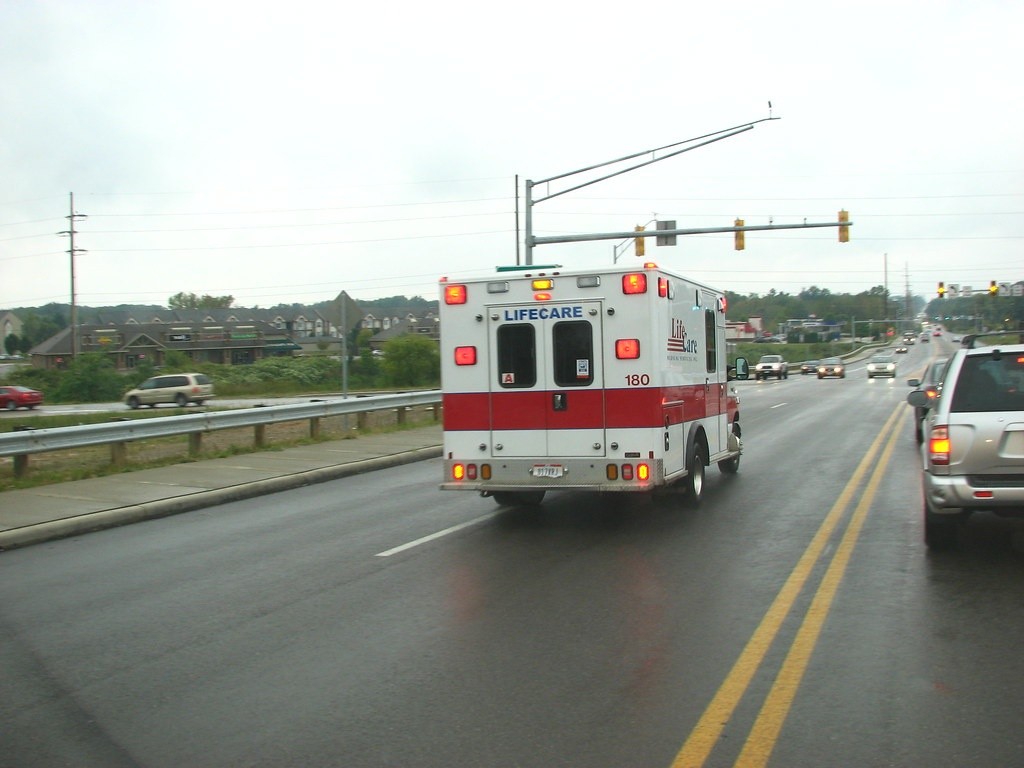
[938,282,944,298]
[989,281,998,296]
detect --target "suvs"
[124,373,216,409]
[755,355,787,380]
[908,330,1023,552]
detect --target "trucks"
[437,266,750,510]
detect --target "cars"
[801,360,820,375]
[753,335,781,344]
[896,345,908,354]
[905,358,949,445]
[0,385,44,411]
[952,335,961,342]
[920,321,943,343]
[866,356,897,380]
[0,354,24,361]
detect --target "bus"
[903,332,915,346]
[817,357,845,379]
